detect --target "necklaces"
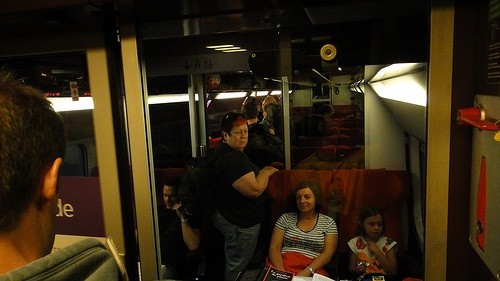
[365,235,370,242]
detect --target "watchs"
[307,265,315,275]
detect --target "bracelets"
[180,218,189,222]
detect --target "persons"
[203,94,363,170]
[264,178,339,281]
[0,69,122,281]
[160,177,207,280]
[345,202,398,280]
[202,112,279,281]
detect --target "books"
[263,266,296,281]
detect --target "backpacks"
[179,147,260,229]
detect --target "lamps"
[368,62,427,107]
[147,93,199,104]
[47,96,93,112]
[215,90,292,99]
[350,79,365,93]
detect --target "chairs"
[91,103,409,281]
